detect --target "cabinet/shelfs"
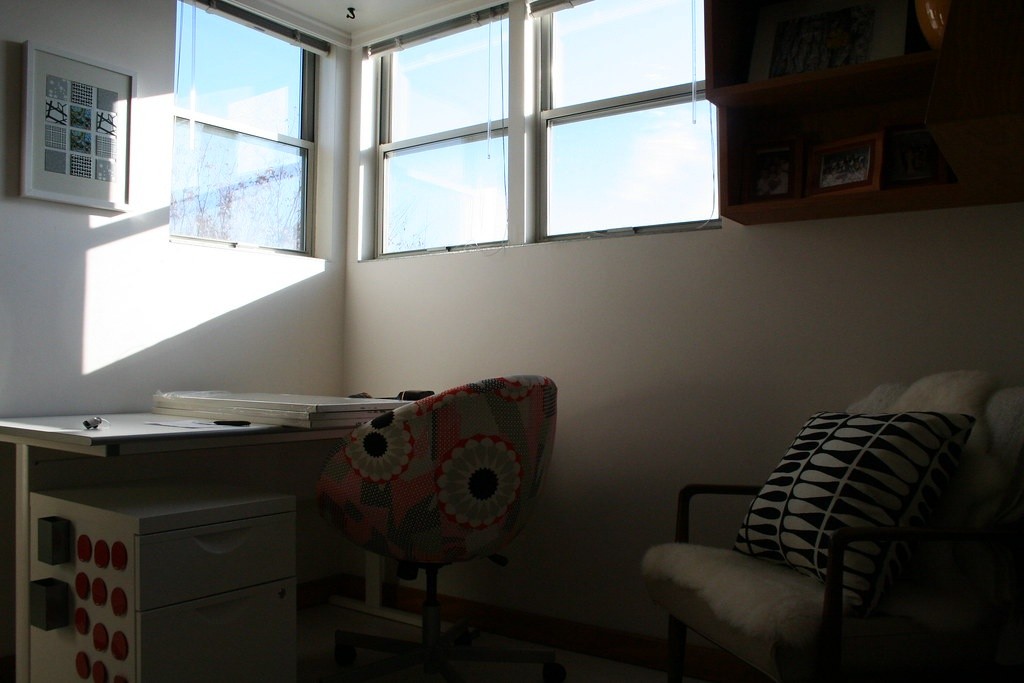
[704,0,1024,226]
[30,473,301,683]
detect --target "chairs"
[315,376,569,683]
[642,371,1024,683]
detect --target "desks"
[0,416,482,683]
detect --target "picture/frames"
[741,133,802,200]
[18,41,138,214]
[806,130,884,196]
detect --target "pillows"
[735,409,979,615]
